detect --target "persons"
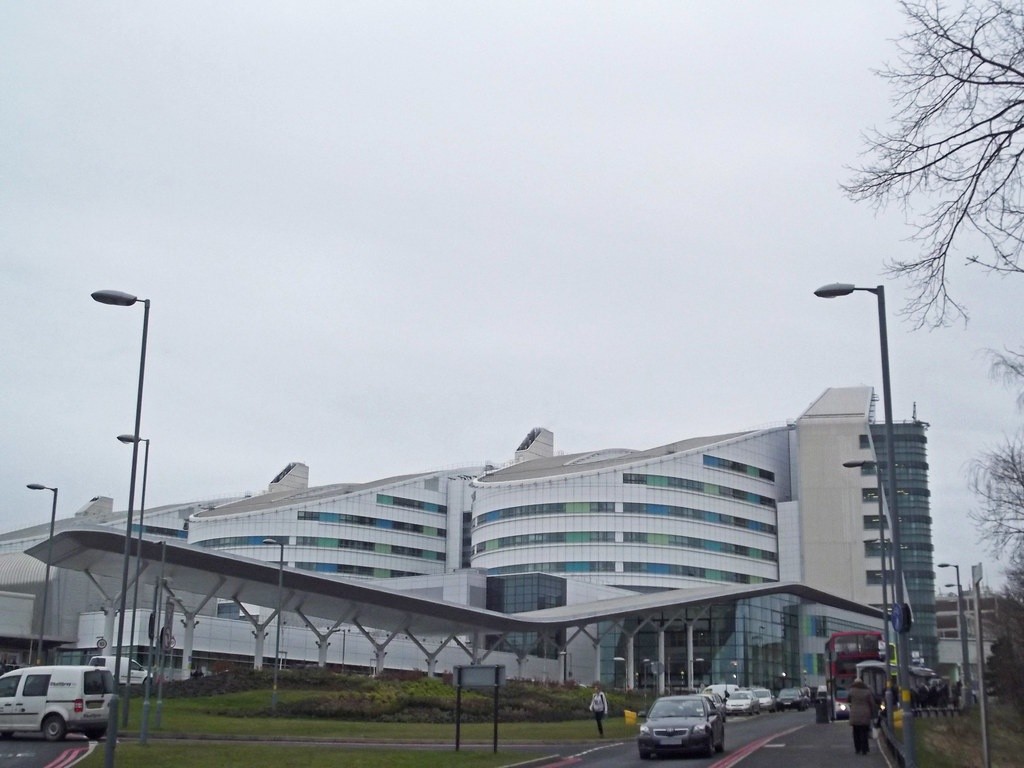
[910,679,963,719]
[847,677,879,756]
[589,685,608,738]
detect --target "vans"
[0,664,116,742]
[86,656,154,689]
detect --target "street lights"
[263,537,286,692]
[813,281,921,768]
[841,460,895,754]
[91,289,151,768]
[937,564,972,712]
[944,582,971,712]
[151,538,168,667]
[27,484,58,665]
[116,433,151,734]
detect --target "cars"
[725,689,761,716]
[753,688,775,713]
[636,694,725,760]
[681,693,726,723]
[776,686,812,712]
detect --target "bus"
[821,630,900,722]
